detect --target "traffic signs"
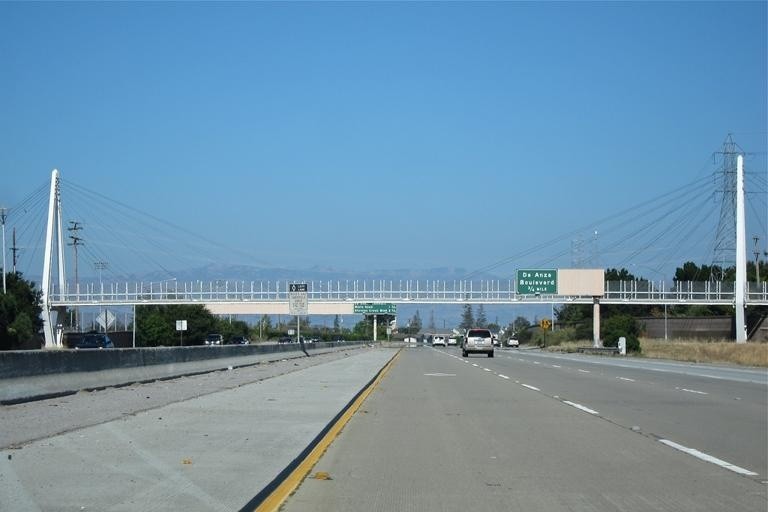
[516,269,556,294]
[353,303,396,314]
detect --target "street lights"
[0,208,9,295]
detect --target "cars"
[203,332,345,345]
[432,334,518,347]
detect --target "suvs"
[75,334,116,349]
[462,328,495,359]
